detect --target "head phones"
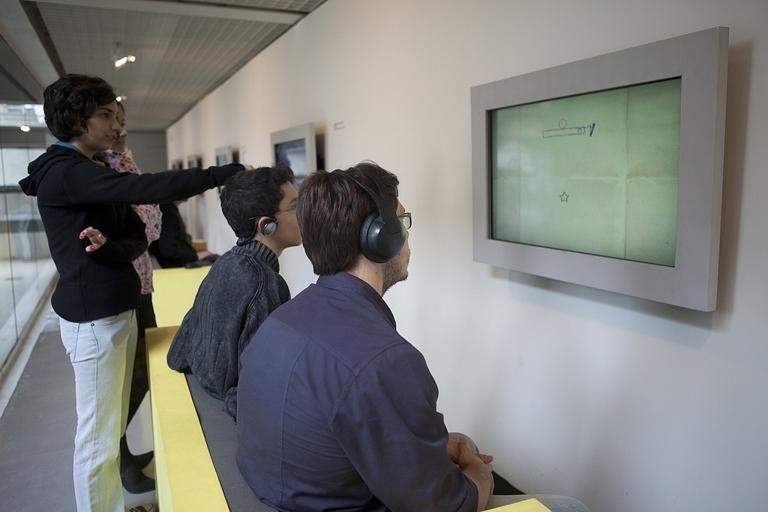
[236,216,279,246]
[336,164,406,263]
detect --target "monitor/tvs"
[472,26,728,313]
[172,160,180,169]
[214,144,234,167]
[187,154,200,169]
[270,122,317,191]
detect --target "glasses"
[397,213,412,229]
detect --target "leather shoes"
[121,450,155,494]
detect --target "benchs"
[146,321,548,512]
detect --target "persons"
[94,98,166,495]
[164,160,303,422]
[150,197,210,267]
[18,73,254,512]
[234,159,525,511]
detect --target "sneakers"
[130,503,155,512]
